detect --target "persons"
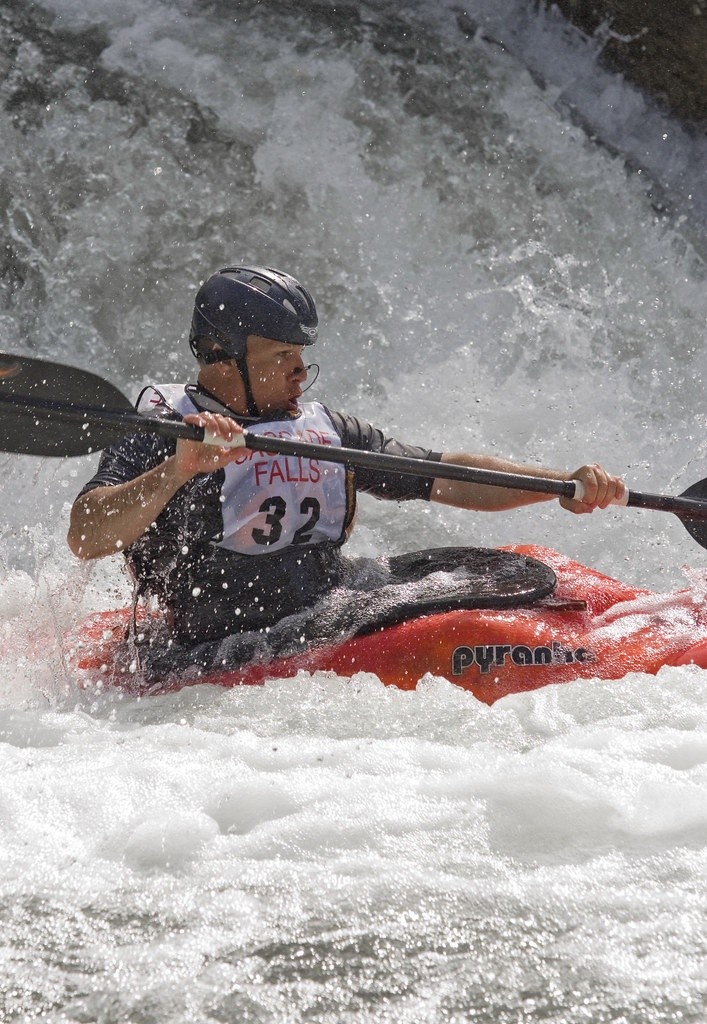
[68,265,625,649]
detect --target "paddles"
[0,353,707,549]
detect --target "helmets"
[189,264,318,363]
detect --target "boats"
[62,546,701,707]
